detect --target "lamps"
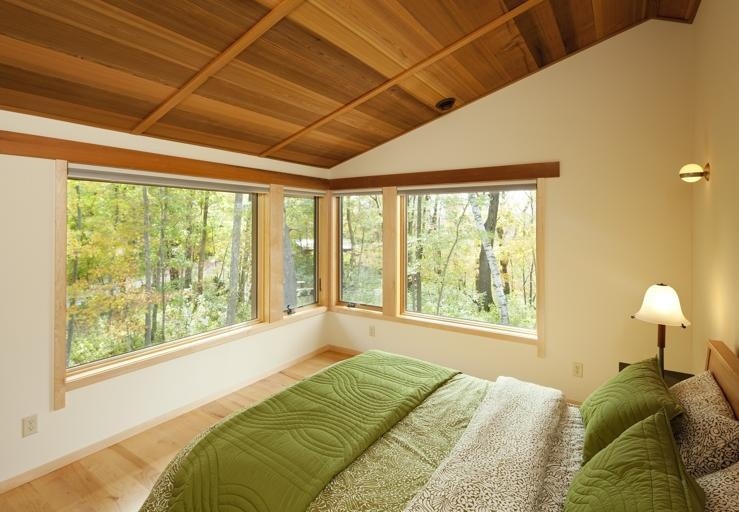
[676,160,713,184]
[631,280,694,372]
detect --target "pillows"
[561,406,708,511]
[689,455,739,512]
[575,352,685,465]
[665,362,739,473]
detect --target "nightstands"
[617,359,698,387]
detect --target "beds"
[132,337,738,512]
